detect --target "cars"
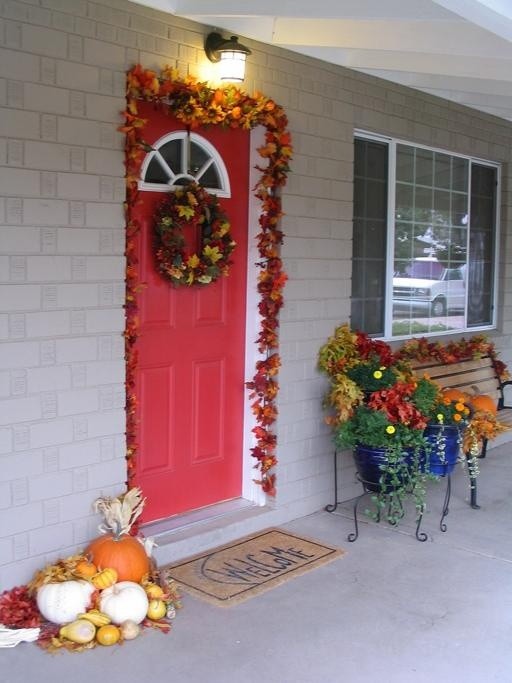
[392,256,466,316]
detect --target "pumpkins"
[36,521,167,646]
[442,385,497,417]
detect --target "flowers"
[150,180,237,290]
[328,377,482,526]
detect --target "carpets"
[156,526,348,610]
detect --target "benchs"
[354,342,512,458]
